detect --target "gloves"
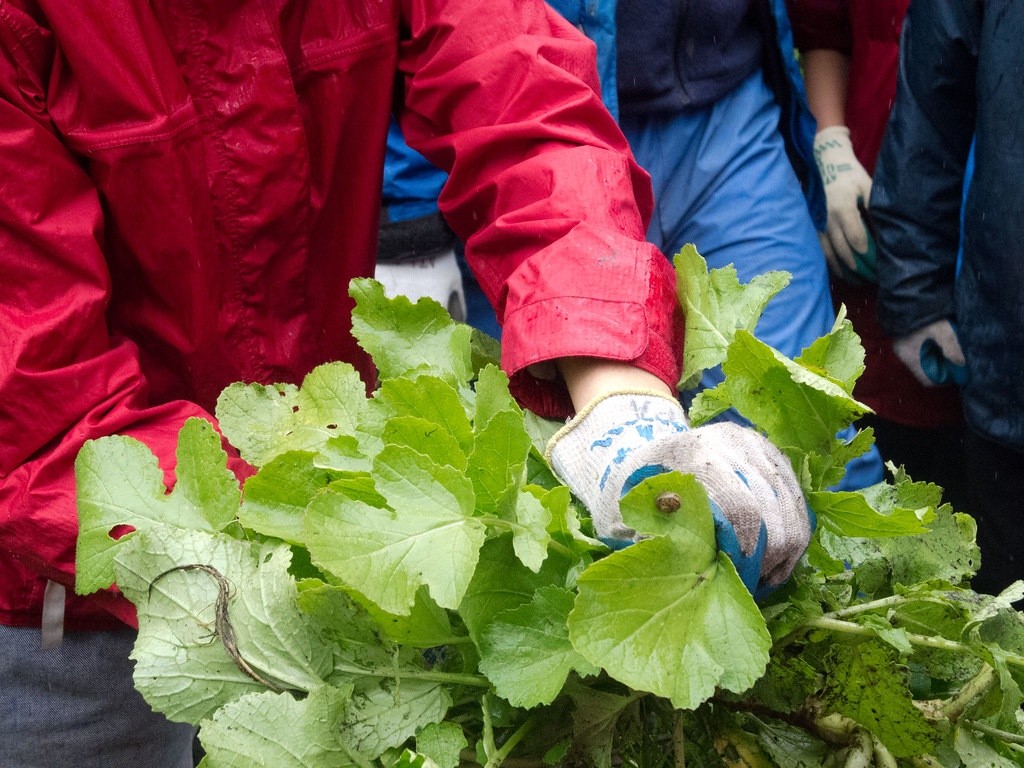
[546,390,811,604]
[813,125,873,279]
[374,248,466,323]
[893,319,966,386]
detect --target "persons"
[0,0,811,768]
[867,0,1024,613]
[373,0,885,534]
[786,0,928,430]
